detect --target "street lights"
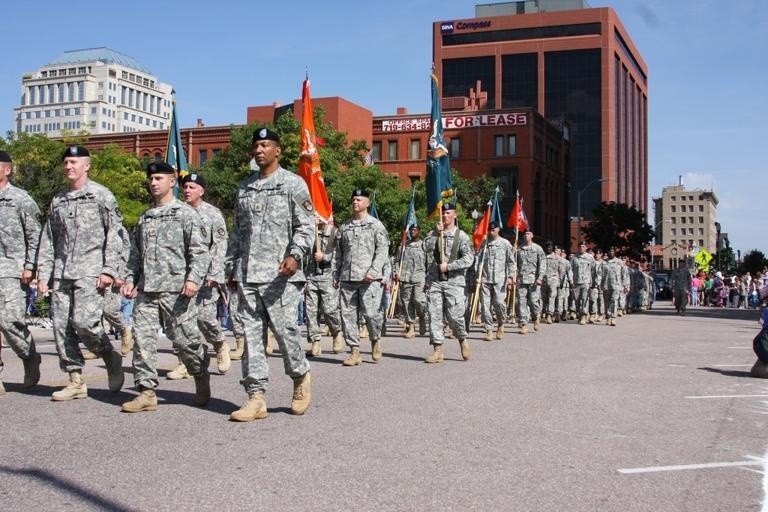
[577,176,609,254]
[723,236,730,271]
[714,222,721,271]
[737,249,741,271]
[650,220,674,263]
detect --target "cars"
[652,278,666,298]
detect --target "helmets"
[351,189,369,198]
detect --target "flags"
[294,80,335,227]
[162,100,191,201]
[427,71,455,223]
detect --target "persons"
[225,147,657,411]
[0,146,228,411]
[223,127,318,424]
[670,256,768,317]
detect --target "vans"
[654,273,670,282]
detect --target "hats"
[253,128,279,142]
[0,150,10,162]
[409,224,420,230]
[489,221,499,229]
[147,161,205,189]
[546,240,615,254]
[62,146,90,161]
[442,203,455,210]
